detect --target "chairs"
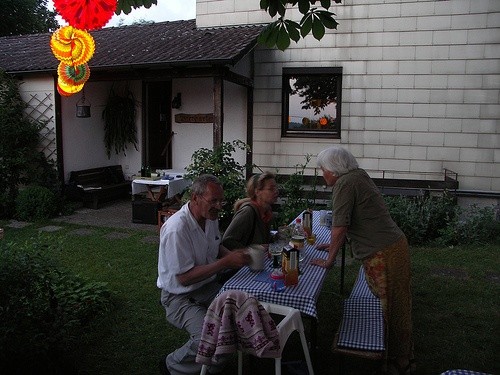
[201,288,313,375]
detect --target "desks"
[131,168,198,222]
[217,209,346,350]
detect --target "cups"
[269,268,284,291]
[151,173,158,181]
[243,243,264,272]
[267,242,284,256]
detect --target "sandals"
[409,359,417,373]
[373,359,411,375]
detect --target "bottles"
[290,218,306,262]
[141,163,146,177]
[145,162,151,178]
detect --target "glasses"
[198,194,226,207]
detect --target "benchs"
[157,201,185,232]
[331,263,389,361]
[69,165,132,209]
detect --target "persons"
[157,175,253,375]
[221,171,278,251]
[311,146,416,375]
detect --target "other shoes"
[159,352,172,375]
